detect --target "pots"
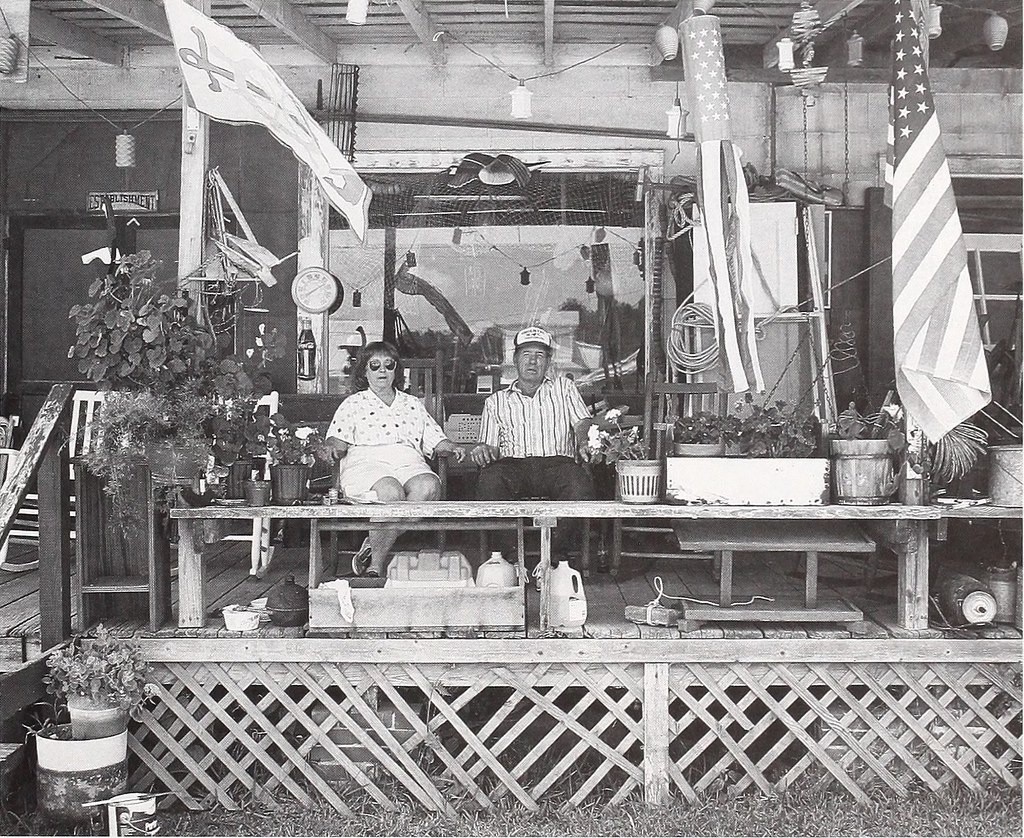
[265,573,309,628]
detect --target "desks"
[162,503,1023,635]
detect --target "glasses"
[366,360,397,371]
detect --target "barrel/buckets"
[986,444,1023,509]
[547,559,587,629]
[108,793,158,836]
[474,551,517,588]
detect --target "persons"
[316,342,466,578]
[469,327,602,591]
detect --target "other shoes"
[364,564,382,577]
[352,536,374,576]
[514,561,529,584]
[532,563,553,591]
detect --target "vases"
[615,459,664,503]
[268,463,313,504]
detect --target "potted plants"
[672,413,742,456]
[23,700,129,821]
[44,624,160,741]
[744,396,822,458]
[69,382,244,537]
[244,454,270,507]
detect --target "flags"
[163,0,373,245]
[883,0,992,445]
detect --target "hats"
[514,325,553,353]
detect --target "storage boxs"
[662,455,830,507]
[306,567,525,628]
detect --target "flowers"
[245,413,323,465]
[588,409,651,462]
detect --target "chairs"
[326,349,444,576]
[477,447,591,582]
[609,371,728,576]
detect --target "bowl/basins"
[222,609,261,631]
[250,597,271,622]
